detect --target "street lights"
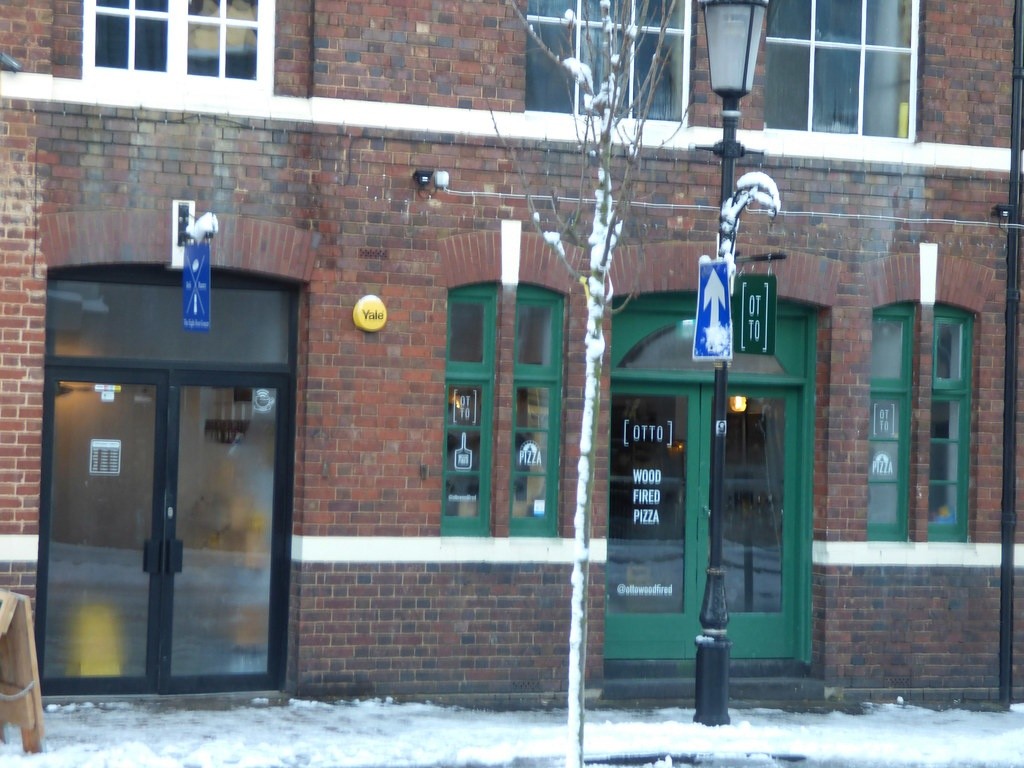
[692,1,773,731]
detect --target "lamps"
[990,203,1015,218]
[410,169,433,186]
[0,51,24,72]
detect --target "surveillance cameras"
[197,212,219,238]
